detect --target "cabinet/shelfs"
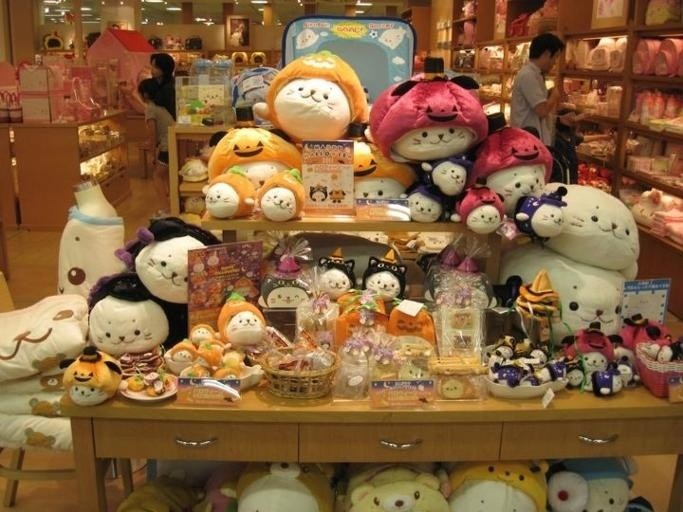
[0,109,131,233]
[399,6,429,55]
[450,0,681,319]
[167,124,233,219]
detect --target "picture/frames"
[225,14,252,52]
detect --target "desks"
[59,382,682,512]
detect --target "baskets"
[636,343,682,398]
[261,347,340,398]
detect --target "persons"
[230,24,243,48]
[510,32,565,149]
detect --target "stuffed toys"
[0,51,681,510]
[1,50,683,511]
